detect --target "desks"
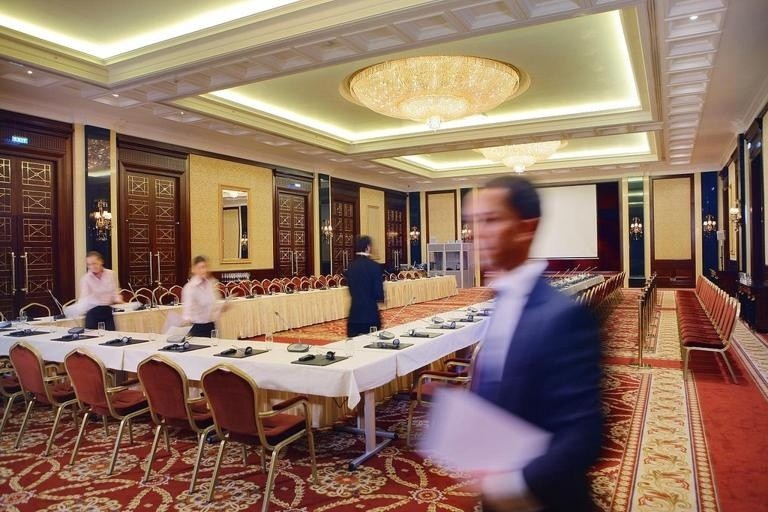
[124,328,392,472]
[322,272,602,440]
[1,320,161,422]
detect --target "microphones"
[0,261,598,361]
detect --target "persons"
[343,236,387,338]
[179,253,233,338]
[75,249,125,332]
[453,172,611,510]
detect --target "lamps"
[729,208,743,232]
[629,217,644,242]
[409,226,421,246]
[90,199,112,241]
[347,57,521,129]
[702,213,718,240]
[322,219,334,245]
[472,139,568,173]
[462,223,473,240]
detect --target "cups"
[253,289,257,298]
[19,315,28,329]
[145,298,151,309]
[98,322,105,336]
[174,296,179,306]
[272,288,275,296]
[370,325,378,347]
[211,329,219,346]
[265,329,273,352]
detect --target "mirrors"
[219,185,253,265]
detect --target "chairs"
[8,340,80,457]
[573,271,626,325]
[0,358,60,437]
[17,271,458,340]
[135,353,216,491]
[406,344,480,447]
[199,362,319,511]
[63,346,150,477]
[673,275,742,384]
